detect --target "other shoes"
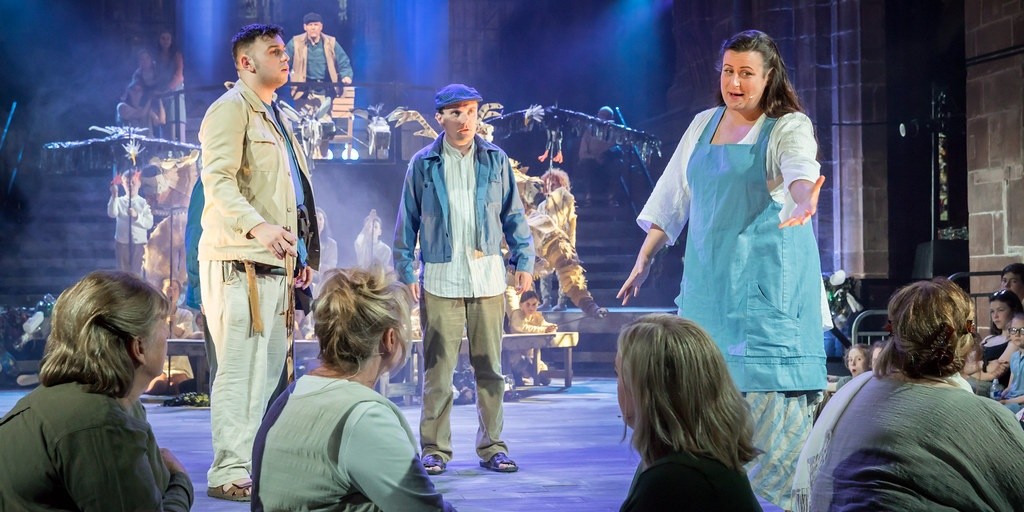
[512,371,524,386]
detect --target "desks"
[163,337,414,410]
[540,306,678,366]
[413,330,580,393]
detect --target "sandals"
[208,479,252,501]
[421,454,446,474]
[480,452,518,472]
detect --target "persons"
[354,209,392,270]
[618,312,763,512]
[392,83,535,475]
[813,340,888,424]
[107,169,153,273]
[116,29,186,158]
[311,207,338,283]
[578,106,623,207]
[964,263,1024,413]
[615,29,827,512]
[505,168,609,386]
[285,12,353,159]
[183,23,321,502]
[810,277,1024,512]
[250,268,456,512]
[142,277,194,395]
[0,269,194,512]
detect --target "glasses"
[993,290,1014,301]
[1008,327,1024,335]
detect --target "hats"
[304,12,322,23]
[435,84,482,109]
